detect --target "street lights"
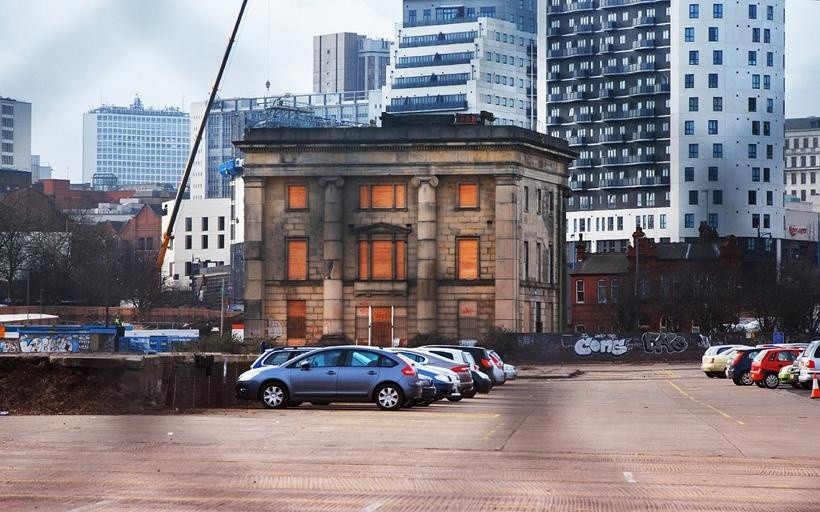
[756,223,777,259]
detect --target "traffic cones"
[809,373,820,399]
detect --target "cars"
[700,340,820,389]
[234,343,518,412]
